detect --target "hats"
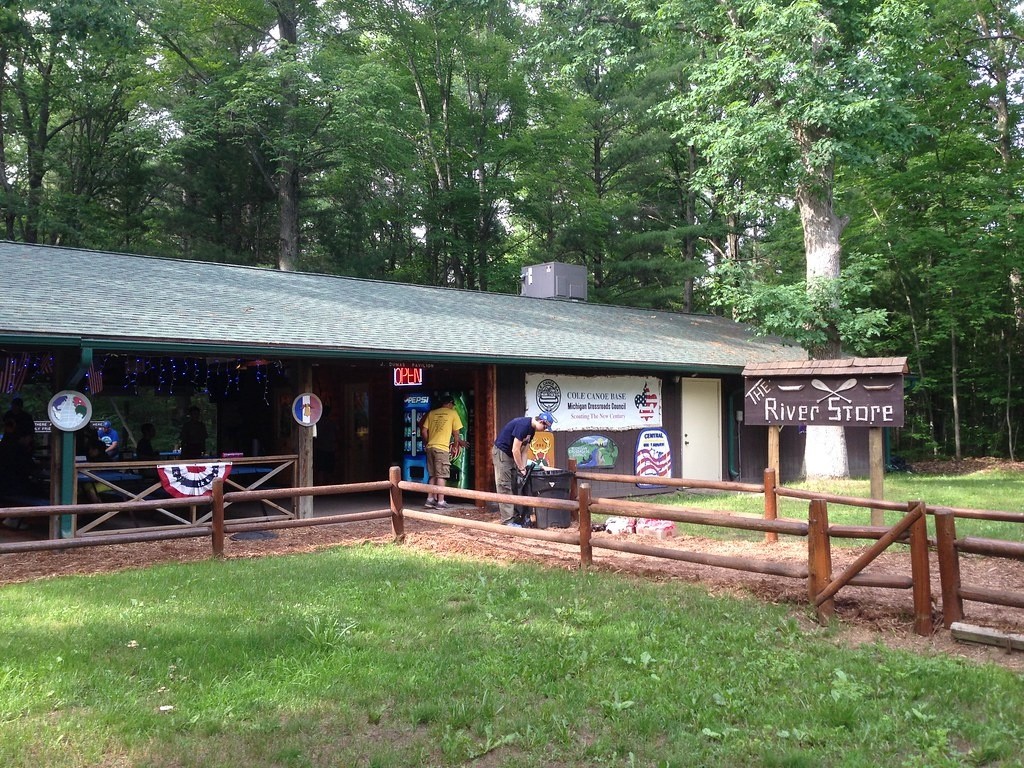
[441,397,456,405]
[102,420,111,429]
[538,412,553,432]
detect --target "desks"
[133,450,181,460]
[29,466,278,522]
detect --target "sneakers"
[424,498,454,510]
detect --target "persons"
[0,397,209,505]
[492,412,554,526]
[418,396,470,510]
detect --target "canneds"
[403,411,427,452]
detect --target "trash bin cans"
[529,469,576,528]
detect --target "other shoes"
[504,518,533,528]
